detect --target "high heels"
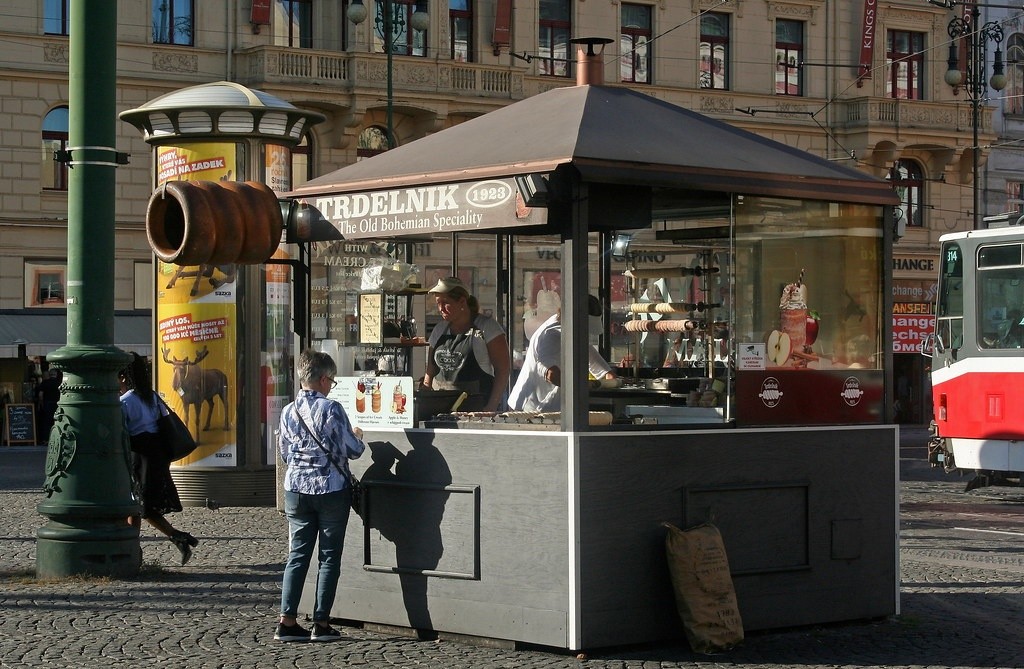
[169,529,198,565]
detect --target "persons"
[274,350,364,641]
[117,351,197,564]
[38,370,60,447]
[422,278,510,413]
[507,294,615,415]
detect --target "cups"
[356,381,365,412]
[782,308,806,353]
[372,385,381,412]
[393,385,402,414]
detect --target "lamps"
[513,173,549,208]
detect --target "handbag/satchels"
[152,389,198,463]
[343,473,363,501]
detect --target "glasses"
[319,375,337,389]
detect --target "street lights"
[945,6,1007,230]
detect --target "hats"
[588,314,604,334]
[428,277,470,300]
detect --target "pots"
[668,378,701,394]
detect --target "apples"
[402,395,405,406]
[768,327,791,365]
[390,402,397,412]
[806,316,819,345]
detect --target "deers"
[166,170,237,296]
[161,343,228,446]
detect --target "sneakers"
[273,621,311,641]
[311,622,342,640]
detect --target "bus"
[919,227,1024,480]
